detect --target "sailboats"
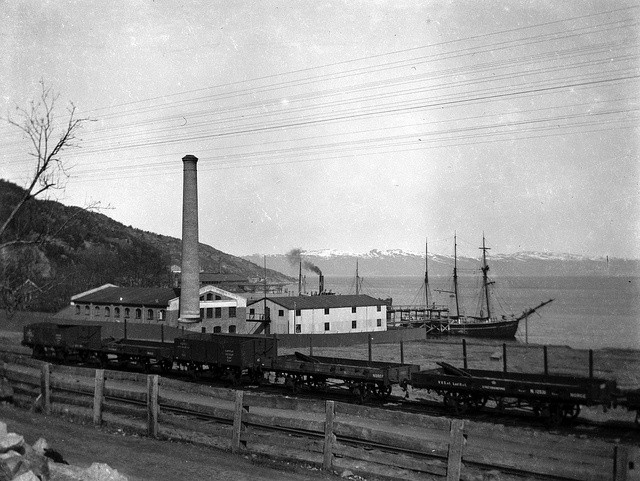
[400,229,555,340]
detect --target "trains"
[22,321,640,445]
